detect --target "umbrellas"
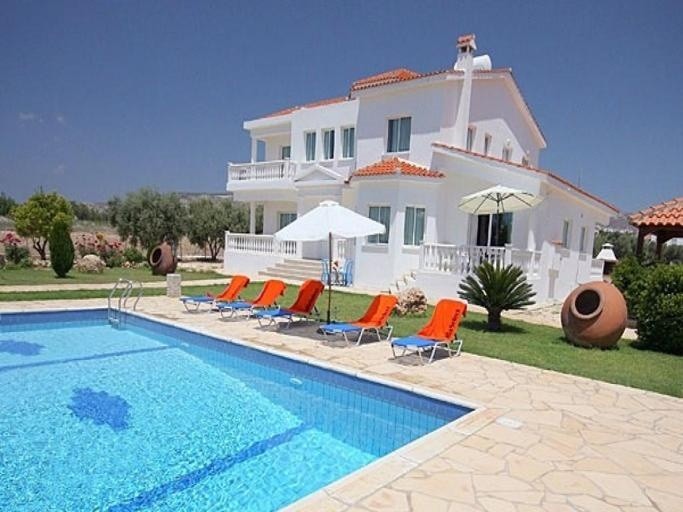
[456,184,545,246]
[274,199,387,325]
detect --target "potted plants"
[456,259,536,328]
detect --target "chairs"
[178,275,249,312]
[390,299,466,366]
[215,279,286,320]
[320,259,353,287]
[253,279,324,331]
[319,294,398,345]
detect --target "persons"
[333,260,343,283]
